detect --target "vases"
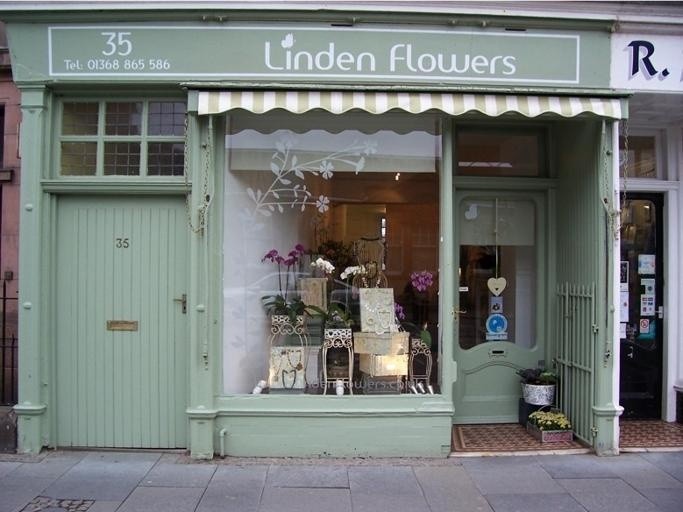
[526,421,573,442]
[331,267,340,289]
[521,383,555,406]
[324,327,352,339]
[271,314,308,328]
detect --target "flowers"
[528,410,572,430]
[323,301,355,328]
[319,239,355,267]
[244,131,378,227]
[408,269,434,292]
[259,243,329,326]
[515,366,557,385]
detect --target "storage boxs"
[352,332,411,355]
[359,353,409,376]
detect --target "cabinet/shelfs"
[268,328,311,394]
[321,340,354,395]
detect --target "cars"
[223,271,369,324]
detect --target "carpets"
[450,422,591,453]
[619,419,683,448]
